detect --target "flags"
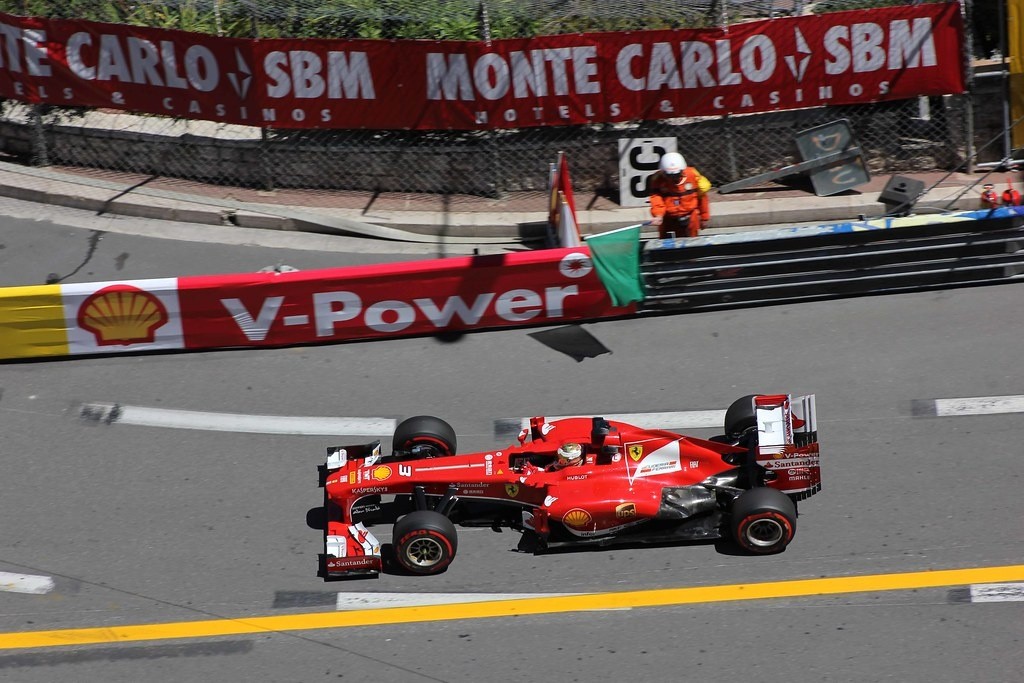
[586,226,646,308]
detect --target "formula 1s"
[326,394,823,578]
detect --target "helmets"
[557,443,582,460]
[660,152,688,186]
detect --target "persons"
[520,442,583,474]
[650,152,710,239]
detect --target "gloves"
[520,461,538,474]
